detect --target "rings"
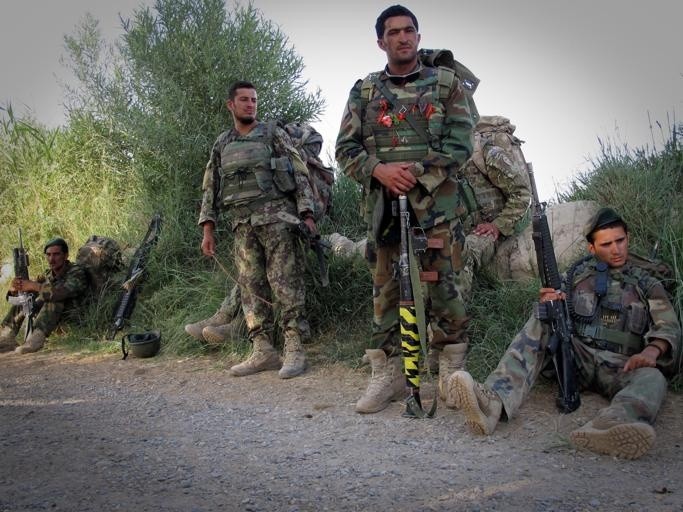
[490,228,494,232]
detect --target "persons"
[185,232,363,351]
[422,116,531,373]
[0,239,95,354]
[198,81,318,380]
[335,3,478,414]
[448,207,682,461]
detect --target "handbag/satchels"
[269,155,297,192]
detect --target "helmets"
[125,328,162,359]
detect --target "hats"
[583,206,624,237]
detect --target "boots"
[437,343,469,401]
[447,370,504,436]
[230,336,282,377]
[15,329,45,354]
[184,310,232,341]
[568,407,657,460]
[426,342,439,374]
[201,319,240,344]
[355,348,407,414]
[278,335,307,379]
[0,330,18,353]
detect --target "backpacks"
[76,235,123,292]
[567,250,677,353]
[357,47,481,161]
[213,122,334,224]
[472,115,535,235]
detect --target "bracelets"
[646,344,662,358]
[304,214,316,225]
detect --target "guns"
[102,215,160,340]
[392,194,444,417]
[525,161,580,412]
[5,227,35,344]
[293,222,333,250]
